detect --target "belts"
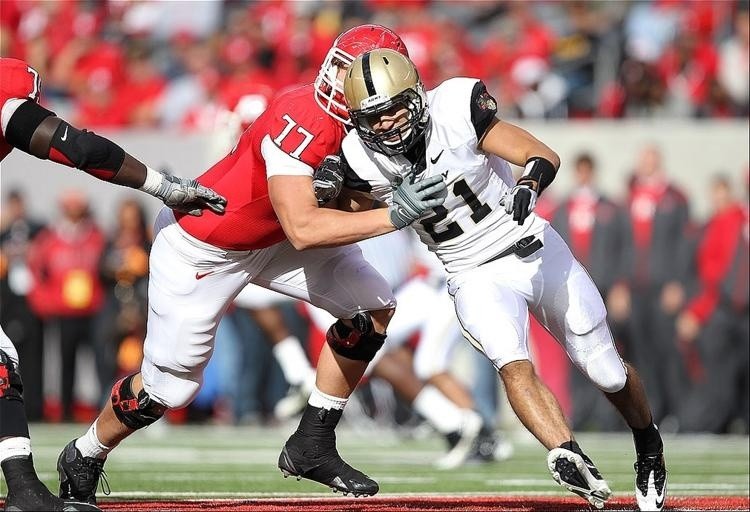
[477,235,544,267]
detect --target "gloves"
[312,152,348,206]
[385,172,449,232]
[498,183,538,226]
[154,169,228,216]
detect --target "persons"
[0,0,748,473]
[58,23,414,511]
[306,48,670,512]
[0,58,230,511]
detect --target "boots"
[277,429,381,498]
[1,469,105,512]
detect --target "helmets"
[342,48,431,154]
[318,24,411,124]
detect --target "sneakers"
[56,437,107,511]
[432,407,484,471]
[546,441,613,510]
[468,408,517,462]
[628,424,668,511]
